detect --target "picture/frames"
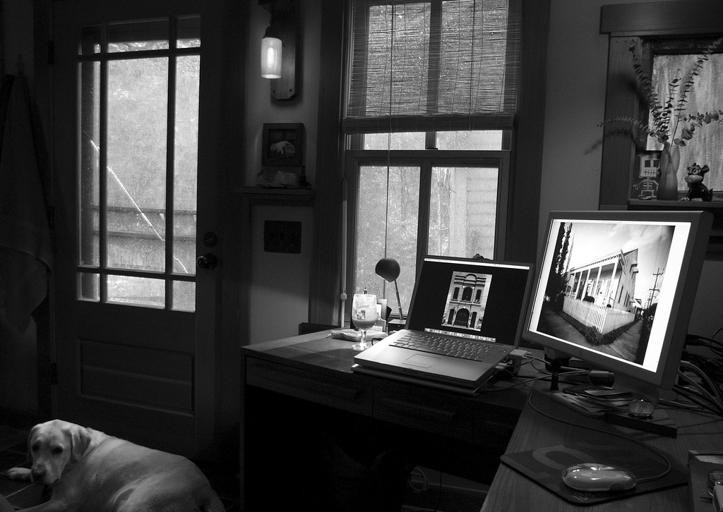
[261,122,304,167]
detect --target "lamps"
[375,259,404,319]
[259,1,304,101]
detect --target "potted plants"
[593,36,723,199]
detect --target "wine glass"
[351,293,378,352]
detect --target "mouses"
[563,462,636,493]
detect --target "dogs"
[6,419,227,512]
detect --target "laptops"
[351,255,536,396]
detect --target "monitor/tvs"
[522,211,715,410]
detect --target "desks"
[240,325,723,512]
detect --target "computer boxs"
[401,466,489,512]
